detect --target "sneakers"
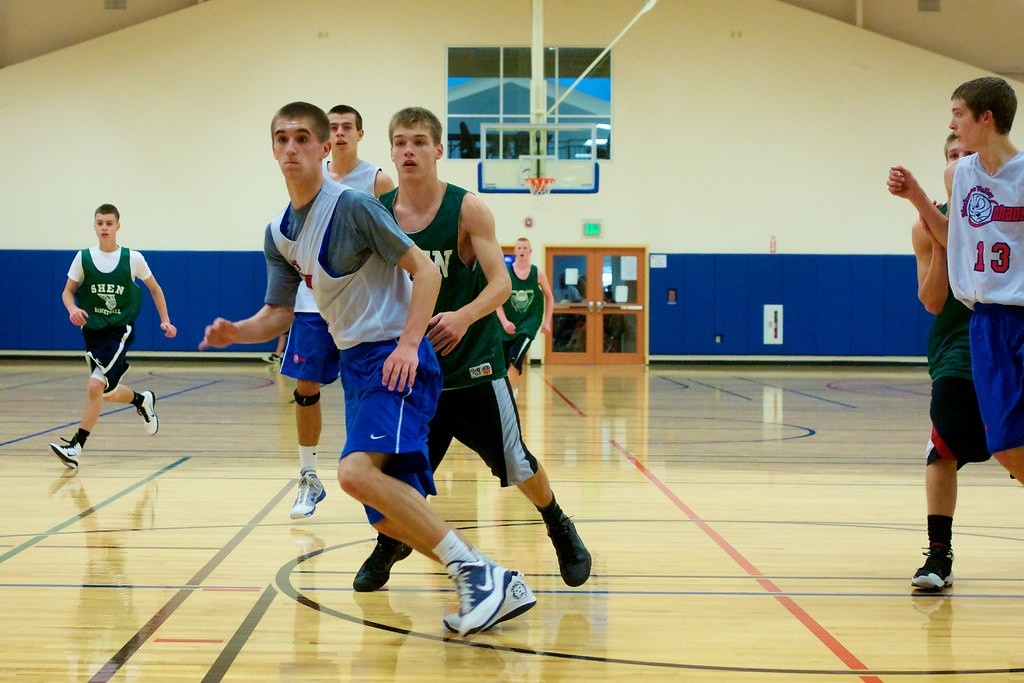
[288,528,326,564]
[136,390,159,436]
[444,571,538,634]
[911,584,955,630]
[352,533,413,592]
[448,547,512,638]
[48,438,83,469]
[545,510,592,587]
[289,475,327,519]
[910,546,954,593]
[49,467,82,499]
[261,356,281,364]
[136,481,158,534]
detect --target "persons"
[888,77,1024,592]
[197,102,625,640]
[49,204,177,469]
